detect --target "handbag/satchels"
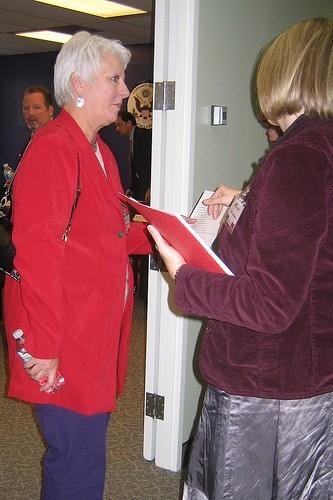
[0,126,81,282]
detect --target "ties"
[127,141,132,188]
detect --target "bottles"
[3,163,14,184]
[12,328,66,396]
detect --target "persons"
[144,17,333,500]
[257,104,286,144]
[1,31,197,500]
[0,83,60,281]
[113,110,155,212]
